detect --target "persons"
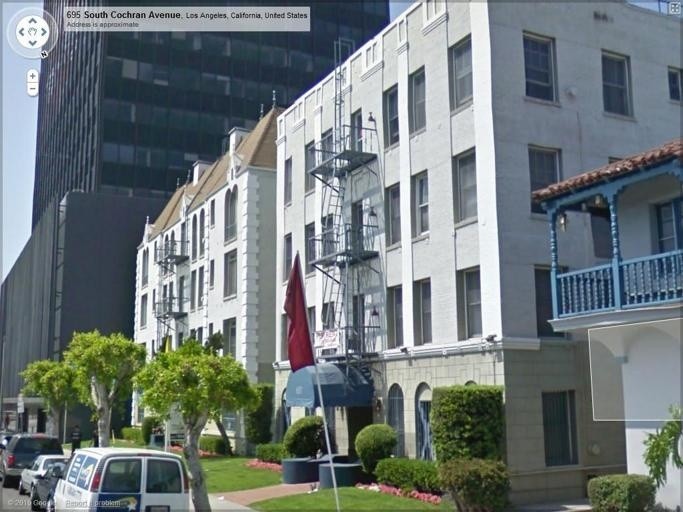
[91,429,99,447]
[70,425,82,454]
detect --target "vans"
[45,446,190,512]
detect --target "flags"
[281,252,316,372]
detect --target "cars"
[0,430,71,512]
